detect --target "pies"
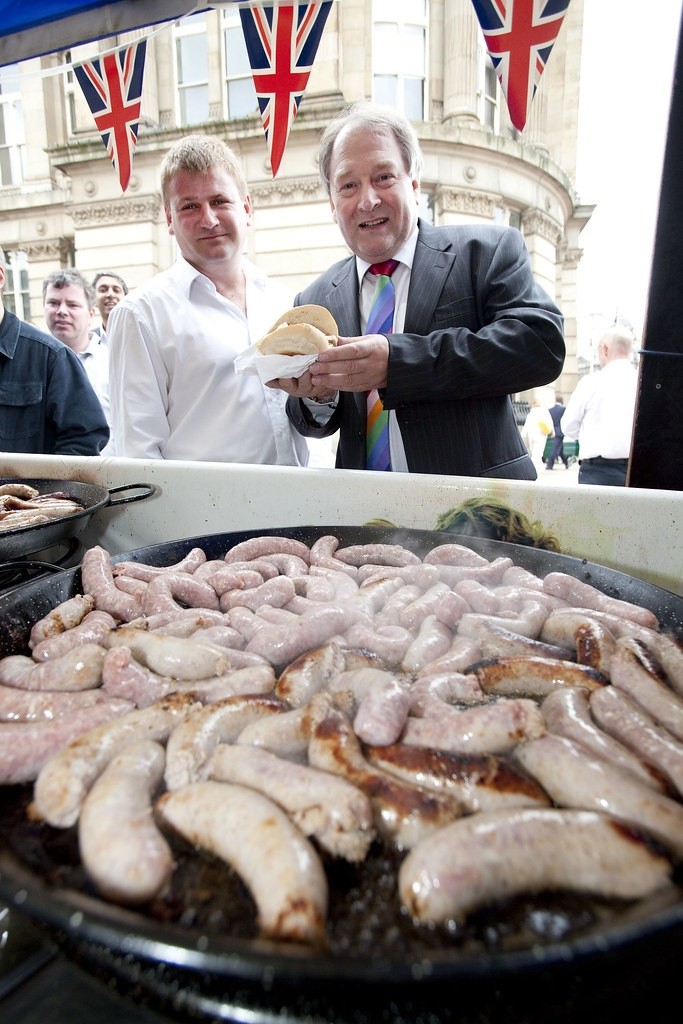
[256,303,338,356]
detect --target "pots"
[0,528,683,1024]
[0,478,158,559]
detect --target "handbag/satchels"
[539,406,551,434]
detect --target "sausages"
[0,532,683,937]
[0,483,87,531]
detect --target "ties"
[364,259,404,471]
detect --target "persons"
[254,106,566,481]
[560,322,639,486]
[521,394,567,476]
[106,134,312,467]
[0,246,128,456]
